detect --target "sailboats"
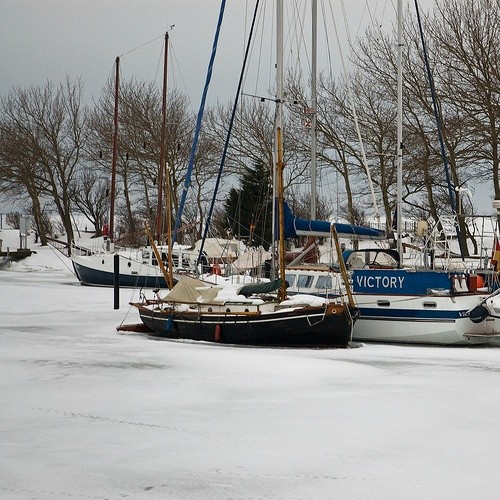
[115,0,361,349]
[71,32,220,288]
[275,0,500,347]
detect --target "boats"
[0,232,32,262]
